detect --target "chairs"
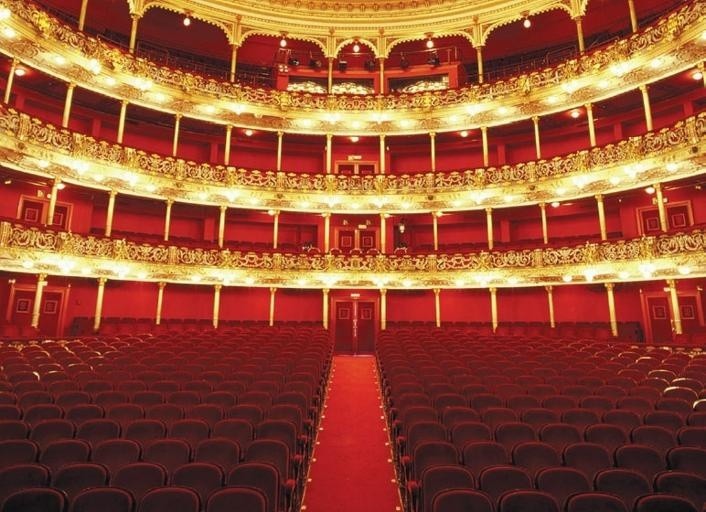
[94,389,127,417]
[562,491,630,512]
[520,407,558,440]
[283,380,321,418]
[171,462,227,491]
[613,442,663,475]
[470,393,502,414]
[387,391,430,427]
[41,370,69,384]
[0,391,19,404]
[246,438,296,512]
[535,465,591,504]
[493,382,524,399]
[538,423,581,452]
[562,440,611,478]
[628,385,662,401]
[401,462,479,511]
[148,380,180,402]
[462,384,490,398]
[93,436,143,471]
[77,418,122,449]
[687,410,706,427]
[631,492,702,512]
[227,461,281,512]
[656,396,692,419]
[384,381,425,396]
[662,386,698,402]
[496,487,561,512]
[584,422,626,451]
[10,369,39,385]
[616,396,653,417]
[72,370,99,385]
[16,381,45,398]
[135,369,162,388]
[84,380,115,399]
[0,486,69,512]
[71,486,136,512]
[205,486,269,512]
[428,382,460,399]
[630,425,676,454]
[541,396,578,416]
[643,410,686,435]
[144,438,192,469]
[124,418,168,463]
[56,391,91,416]
[559,383,593,400]
[267,403,308,483]
[48,315,334,370]
[698,389,706,400]
[526,383,556,402]
[166,390,203,411]
[67,403,106,428]
[0,405,22,421]
[505,396,539,416]
[561,409,599,441]
[461,441,509,476]
[397,422,448,456]
[108,403,145,438]
[102,369,130,390]
[53,462,109,497]
[255,418,304,496]
[132,390,164,409]
[250,380,282,402]
[595,385,626,397]
[692,399,706,413]
[579,397,612,418]
[117,379,146,400]
[394,405,439,435]
[495,422,535,451]
[372,320,706,384]
[0,381,15,392]
[0,439,41,463]
[652,469,706,512]
[205,390,237,412]
[260,370,283,385]
[670,377,705,394]
[442,407,481,436]
[111,462,169,501]
[48,379,82,400]
[227,403,265,429]
[592,466,652,503]
[38,439,92,473]
[190,403,225,429]
[430,487,495,512]
[148,402,185,438]
[213,417,254,462]
[238,390,273,418]
[478,464,535,501]
[170,418,211,462]
[228,369,256,388]
[601,408,643,436]
[676,425,706,448]
[182,379,213,402]
[23,403,64,427]
[29,418,76,452]
[450,422,493,451]
[0,420,30,439]
[275,390,314,452]
[288,371,324,404]
[0,462,52,495]
[198,369,225,388]
[214,379,248,396]
[483,408,516,441]
[137,485,203,511]
[510,441,560,477]
[665,443,706,469]
[399,439,459,491]
[435,394,467,415]
[167,369,193,387]
[17,390,54,418]
[194,437,242,488]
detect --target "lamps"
[424,31,435,49]
[567,107,582,120]
[242,128,256,136]
[519,10,532,29]
[13,66,28,78]
[182,7,196,27]
[352,35,363,54]
[690,70,704,82]
[279,30,290,48]
